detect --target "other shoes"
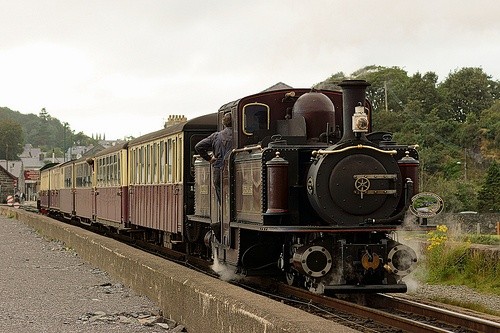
[211,221,222,245]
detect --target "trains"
[36,80,418,293]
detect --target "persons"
[194,112,246,207]
[245,110,267,144]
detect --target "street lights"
[63,121,70,161]
